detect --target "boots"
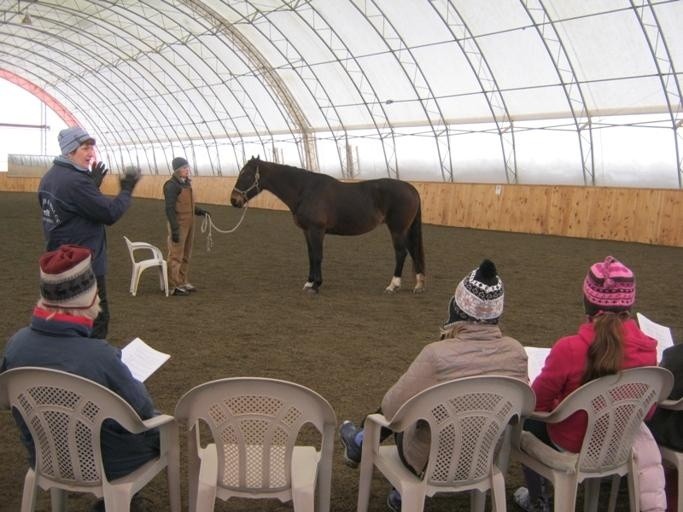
[512,466,550,512]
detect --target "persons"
[37,126,143,340]
[337,259,528,512]
[0,242,163,512]
[645,342,683,454]
[162,156,213,296]
[512,254,657,512]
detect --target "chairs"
[657,395,683,512]
[356,373,536,512]
[173,376,338,511]
[511,366,674,512]
[123,236,169,298]
[0,365,182,512]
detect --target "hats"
[58,127,96,155]
[172,158,188,171]
[442,259,504,328]
[582,257,636,316]
[39,247,97,308]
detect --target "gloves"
[87,161,109,188]
[171,229,179,243]
[119,167,141,193]
[198,208,211,219]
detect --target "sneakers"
[184,283,198,292]
[169,288,189,296]
[339,420,362,469]
[387,489,401,512]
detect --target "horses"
[230,154,426,293]
